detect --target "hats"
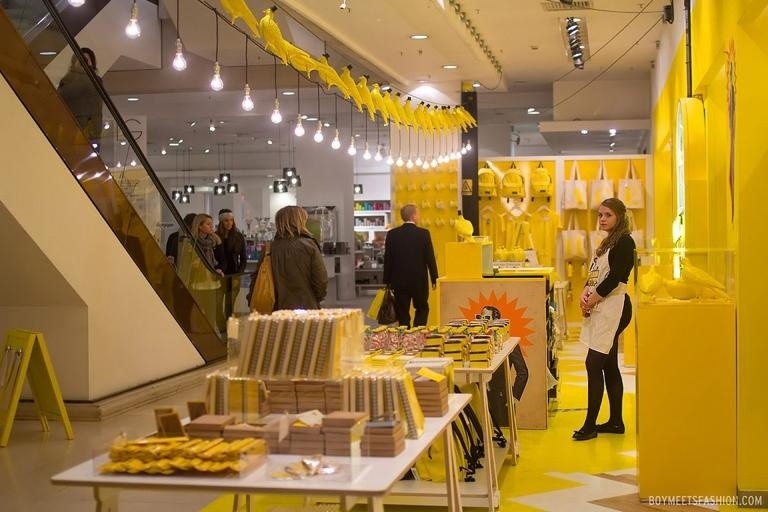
[218,208,234,222]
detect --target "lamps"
[270,119,301,195]
[169,148,199,204]
[566,17,585,72]
[209,139,240,197]
[70,0,476,169]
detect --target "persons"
[245,205,329,314]
[172,276,194,333]
[569,196,637,441]
[181,214,226,330]
[214,209,249,343]
[109,212,150,283]
[167,210,198,271]
[382,204,439,328]
[57,45,108,159]
[473,304,529,429]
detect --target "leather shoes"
[597,423,626,433]
[572,424,599,441]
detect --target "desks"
[369,335,519,508]
[51,392,473,509]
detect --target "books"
[151,304,449,459]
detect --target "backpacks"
[415,382,507,483]
[530,161,553,203]
[501,162,527,203]
[477,162,497,201]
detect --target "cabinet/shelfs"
[352,200,390,251]
[633,246,738,501]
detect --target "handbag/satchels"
[248,242,275,315]
[591,160,614,211]
[628,212,645,249]
[618,159,644,210]
[377,288,399,325]
[590,215,610,257]
[563,160,588,212]
[366,287,387,321]
[561,212,588,263]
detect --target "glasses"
[475,314,492,321]
